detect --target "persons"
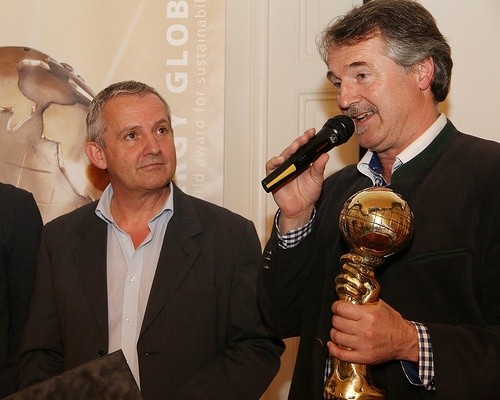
[42,81,286,400]
[1,181,54,400]
[256,0,499,400]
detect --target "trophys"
[322,185,413,400]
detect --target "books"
[0,350,145,400]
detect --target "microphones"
[261,115,356,193]
